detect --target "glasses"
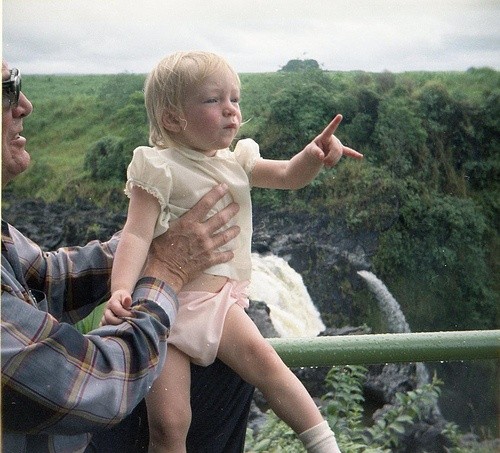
[3,64,24,100]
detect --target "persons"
[1,61,264,453]
[98,48,364,453]
